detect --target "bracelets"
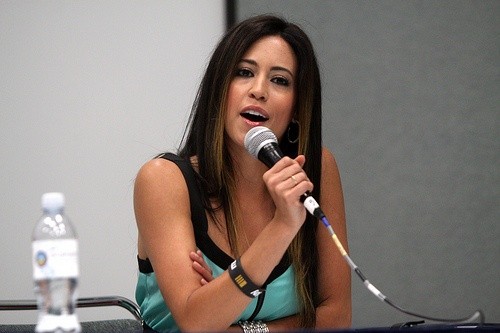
[238,319,269,333]
[228,258,264,297]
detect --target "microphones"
[244,126,326,220]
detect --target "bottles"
[31,193,82,333]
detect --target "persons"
[134,13,353,333]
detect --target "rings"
[291,175,298,185]
